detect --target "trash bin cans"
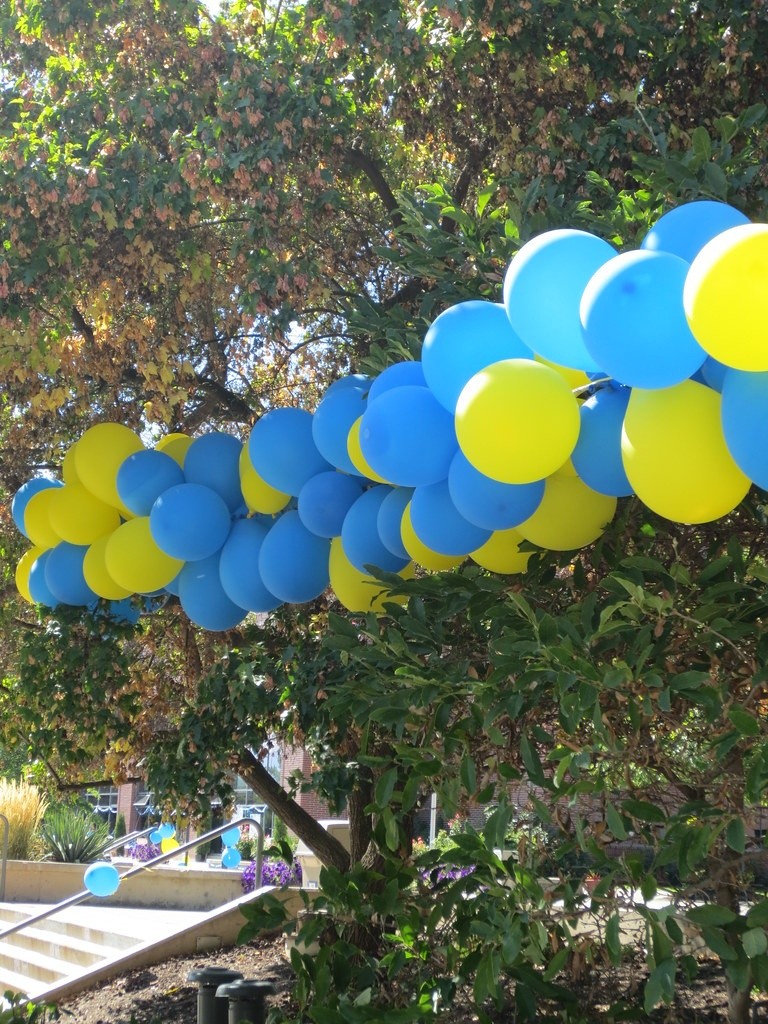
[187,966,244,1024]
[216,978,275,1024]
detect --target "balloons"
[11,201,768,630]
[150,822,180,855]
[84,862,120,896]
[221,827,240,846]
[221,847,241,868]
